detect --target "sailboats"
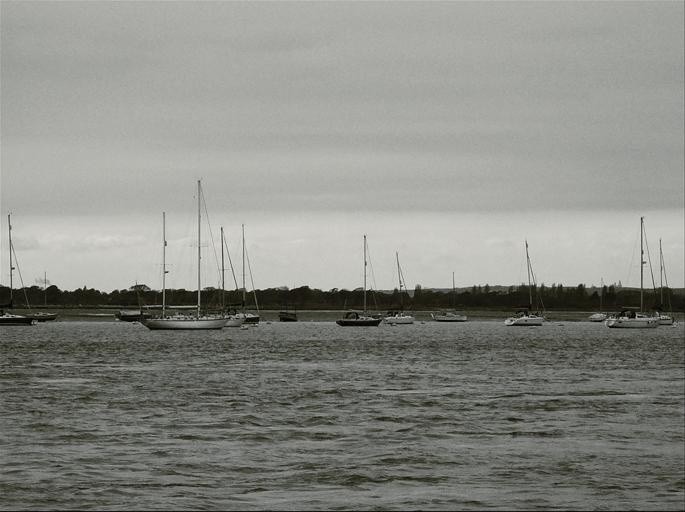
[430,270,470,323]
[653,239,675,325]
[1,213,59,326]
[605,215,659,330]
[588,276,607,322]
[135,177,261,330]
[385,251,417,326]
[355,234,384,319]
[503,237,546,326]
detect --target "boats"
[117,309,151,321]
[276,303,298,322]
